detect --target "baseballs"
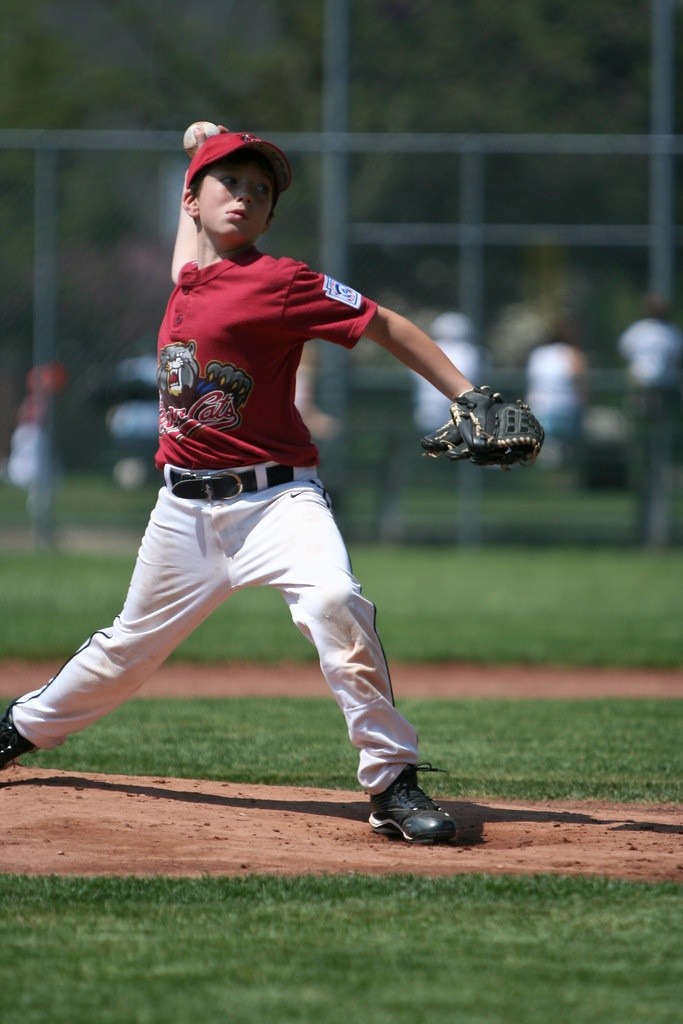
[183,121,222,159]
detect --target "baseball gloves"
[420,384,545,467]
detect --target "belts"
[169,463,294,500]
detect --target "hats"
[186,132,291,211]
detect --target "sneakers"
[368,761,455,843]
[1,701,39,772]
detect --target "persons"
[526,328,587,454]
[411,310,480,448]
[0,125,545,844]
[620,307,683,443]
[6,362,71,551]
